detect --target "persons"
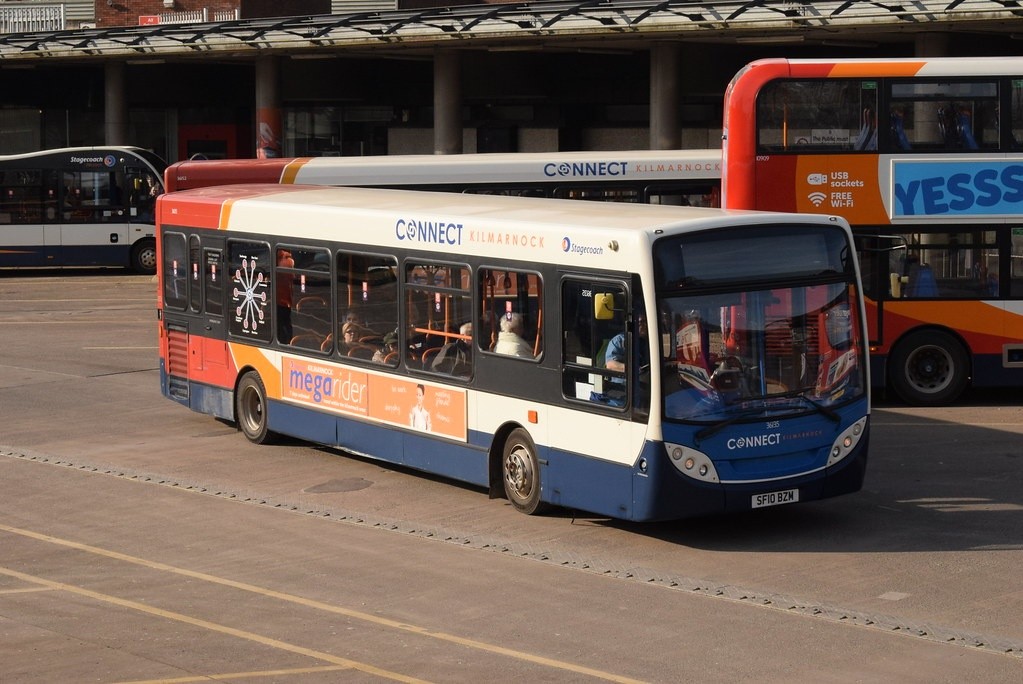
[339,302,422,365]
[432,322,473,378]
[492,313,537,359]
[603,311,654,407]
[276,248,295,345]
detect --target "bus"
[0,144,172,277]
[151,185,888,530]
[161,146,727,211]
[717,52,1023,410]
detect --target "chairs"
[596,339,612,368]
[289,267,498,376]
[852,107,981,153]
[913,263,998,299]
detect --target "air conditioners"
[79,22,96,28]
